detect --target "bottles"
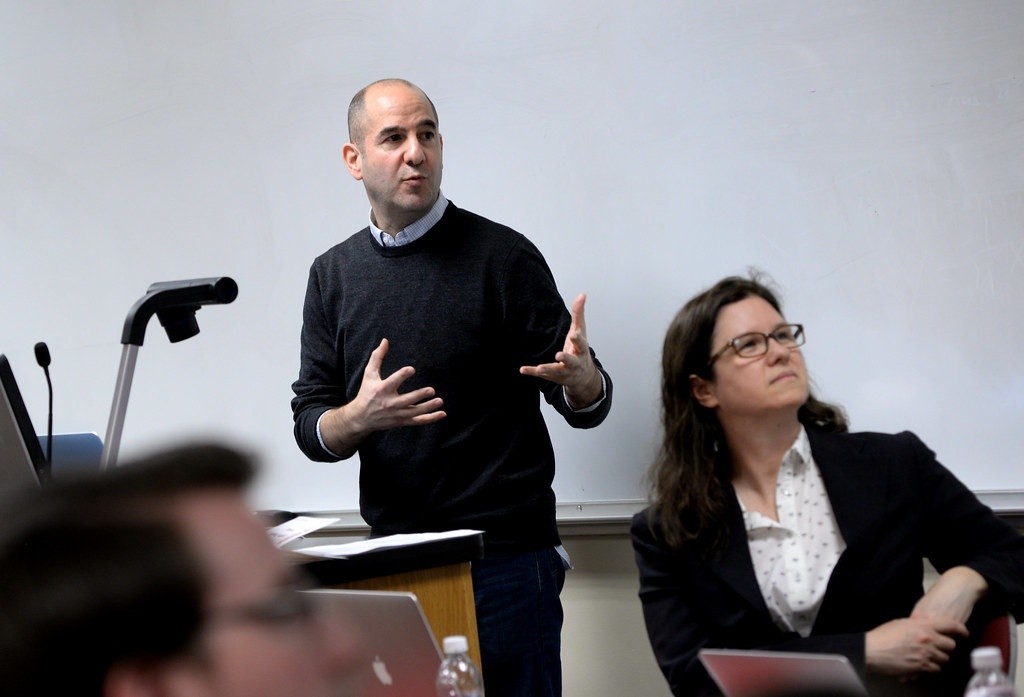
[964,647,1016,697]
[436,635,484,697]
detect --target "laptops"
[698,649,869,697]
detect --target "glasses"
[707,324,806,369]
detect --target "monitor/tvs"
[0,354,50,515]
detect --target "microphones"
[35,342,53,477]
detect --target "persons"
[631,277,1024,697]
[291,81,614,697]
[0,442,343,697]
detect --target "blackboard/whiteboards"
[0,2,1024,534]
